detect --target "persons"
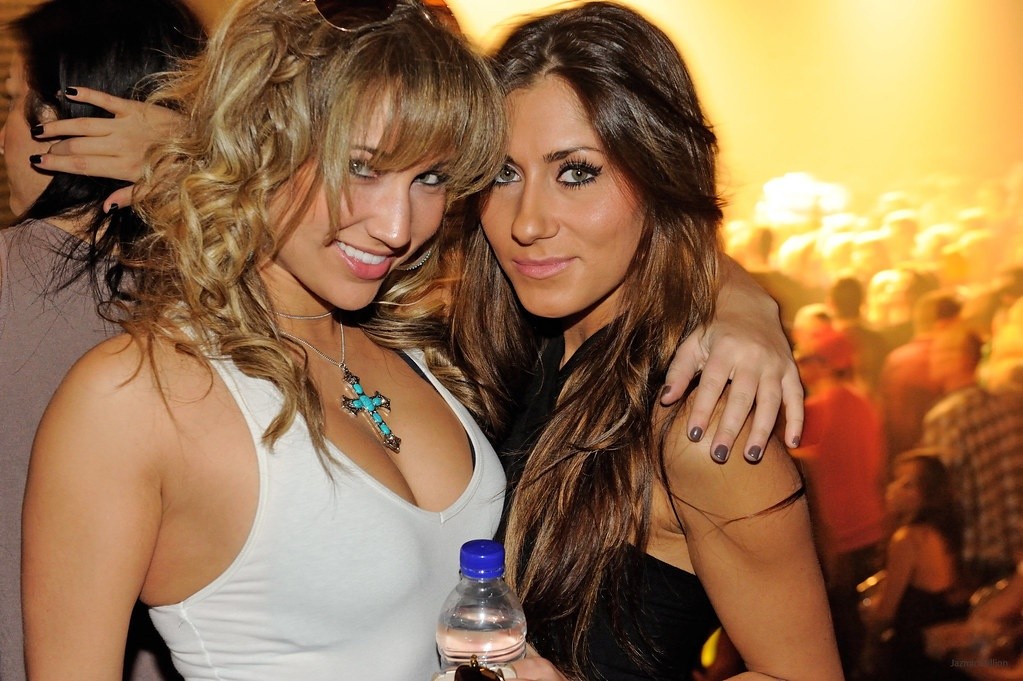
[726,170,1021,605]
[0,0,208,680]
[452,2,845,681]
[19,1,805,681]
[859,447,963,681]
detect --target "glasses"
[300,0,462,38]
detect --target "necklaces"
[268,310,402,453]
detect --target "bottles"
[436,539,527,672]
